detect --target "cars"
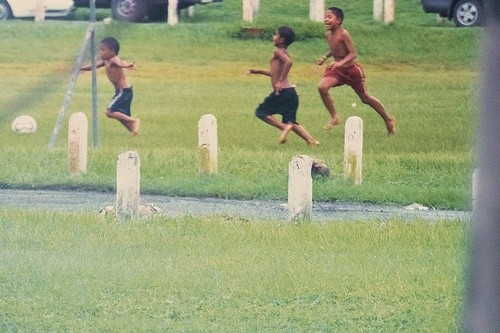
[421,0,484,28]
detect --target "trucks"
[1,0,221,24]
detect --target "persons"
[245,25,320,147]
[317,7,396,139]
[79,37,140,136]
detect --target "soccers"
[12,115,36,135]
